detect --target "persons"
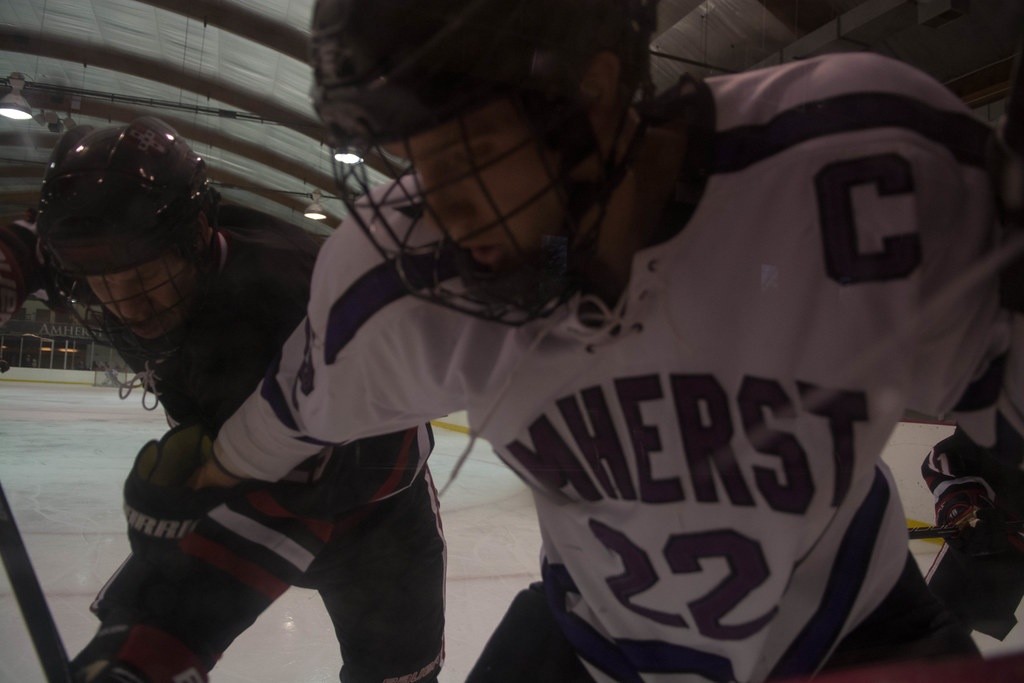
[0,118,448,683]
[124,0,1024,683]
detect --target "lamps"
[0,71,34,121]
[304,138,328,220]
[33,109,77,133]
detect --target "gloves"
[123,421,223,561]
[922,427,1024,580]
[0,219,40,330]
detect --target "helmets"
[308,0,657,325]
[35,122,217,359]
